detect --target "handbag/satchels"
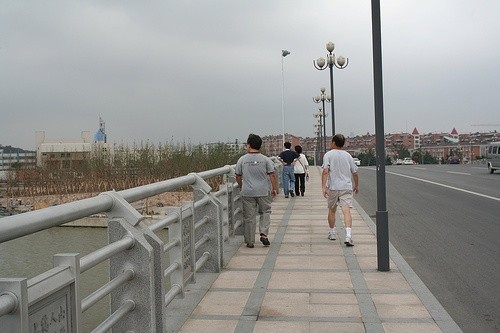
[306,173,309,182]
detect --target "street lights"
[312,86,332,166]
[313,41,350,136]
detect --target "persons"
[321,133,359,246]
[277,141,300,198]
[235,133,276,248]
[294,145,309,197]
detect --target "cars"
[402,157,413,165]
[353,157,361,167]
[448,156,460,164]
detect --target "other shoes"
[246,243,255,248]
[343,236,354,246]
[301,191,304,196]
[260,235,270,245]
[285,194,289,198]
[290,191,294,197]
[327,231,337,240]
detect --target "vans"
[486,141,500,174]
